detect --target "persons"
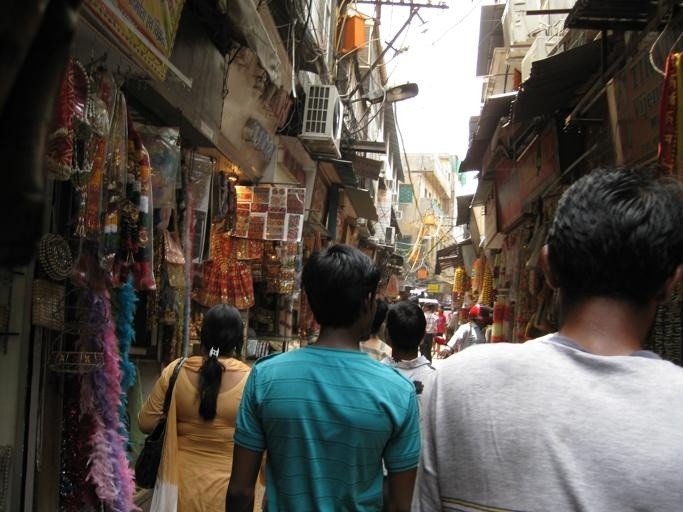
[360,285,490,511]
[223,243,421,511]
[419,164,683,512]
[137,302,266,511]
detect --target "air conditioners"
[302,84,345,160]
[501,0,565,84]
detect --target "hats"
[467,304,491,322]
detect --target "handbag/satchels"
[132,355,188,490]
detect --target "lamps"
[347,80,418,105]
